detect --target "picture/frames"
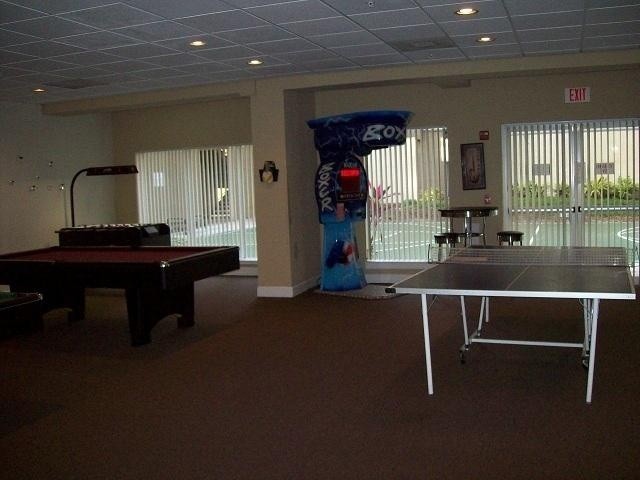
[460,142,487,190]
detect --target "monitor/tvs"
[336,167,361,195]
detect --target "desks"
[438,206,498,248]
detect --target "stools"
[459,233,480,247]
[434,232,457,262]
[498,230,524,246]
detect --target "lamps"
[259,160,278,183]
[69,164,138,227]
